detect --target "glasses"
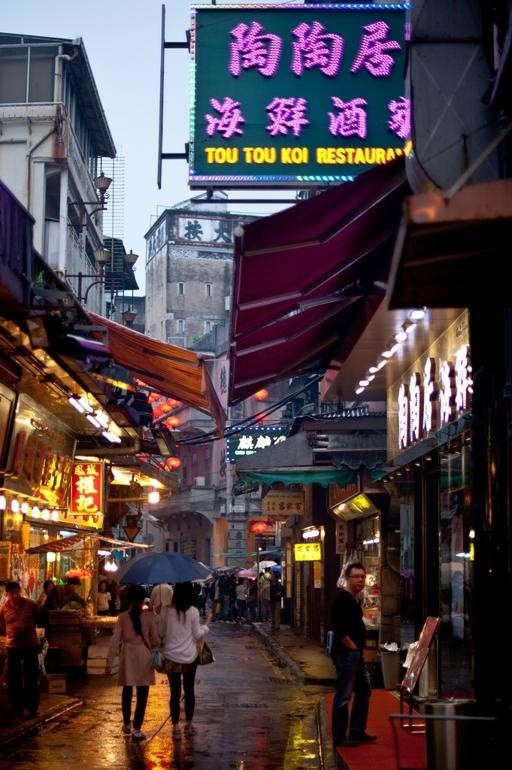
[349,575,367,579]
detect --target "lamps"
[1,326,124,449]
[0,486,61,523]
[352,307,431,403]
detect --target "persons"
[105,583,161,739]
[325,562,378,747]
[44,577,88,617]
[35,581,55,609]
[164,582,213,739]
[1,582,47,718]
[98,559,281,624]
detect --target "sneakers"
[172,729,181,740]
[121,726,131,734]
[132,731,145,741]
[184,726,198,735]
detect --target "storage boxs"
[44,631,121,694]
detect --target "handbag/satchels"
[150,645,165,674]
[196,634,214,666]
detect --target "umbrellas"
[114,550,212,583]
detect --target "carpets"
[325,687,429,770]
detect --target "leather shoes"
[333,736,359,746]
[349,734,377,742]
